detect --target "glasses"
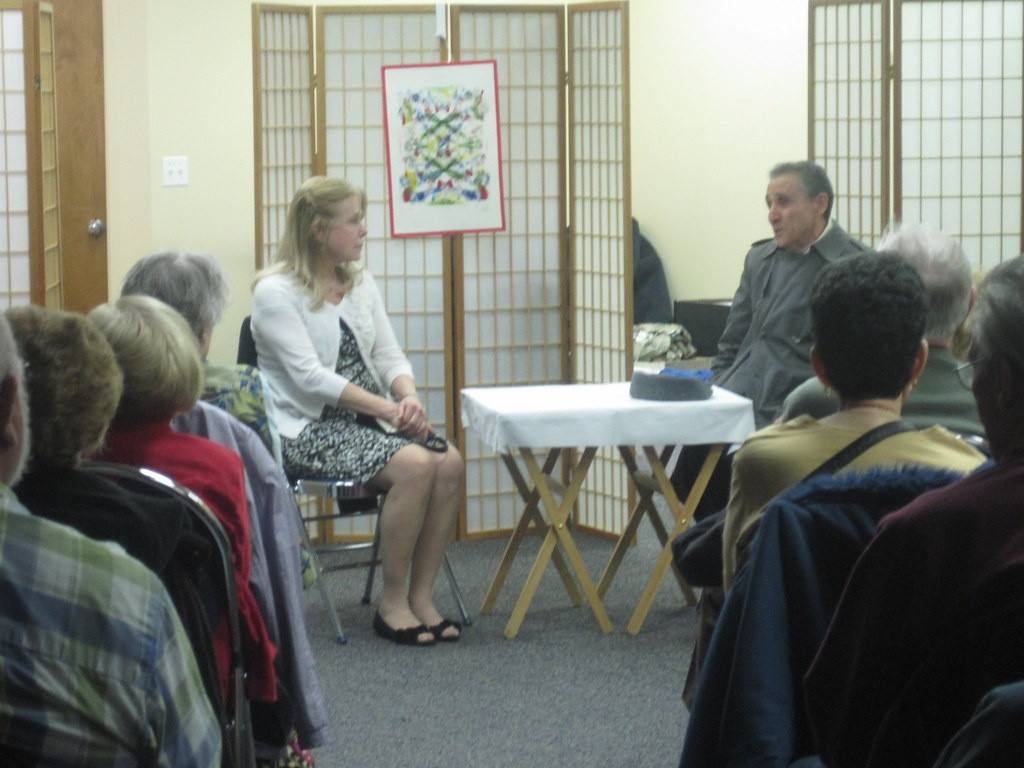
[955,351,995,389]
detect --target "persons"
[630,216,672,324]
[2,305,231,739]
[722,247,989,597]
[250,176,463,648]
[0,312,225,768]
[675,161,880,520]
[768,224,991,452]
[121,248,316,592]
[83,293,278,710]
[802,252,1024,768]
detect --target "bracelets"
[396,392,418,402]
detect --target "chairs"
[82,460,258,767]
[678,462,963,768]
[170,400,329,749]
[236,315,474,645]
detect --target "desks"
[459,381,757,639]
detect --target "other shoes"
[429,618,463,641]
[373,606,437,646]
